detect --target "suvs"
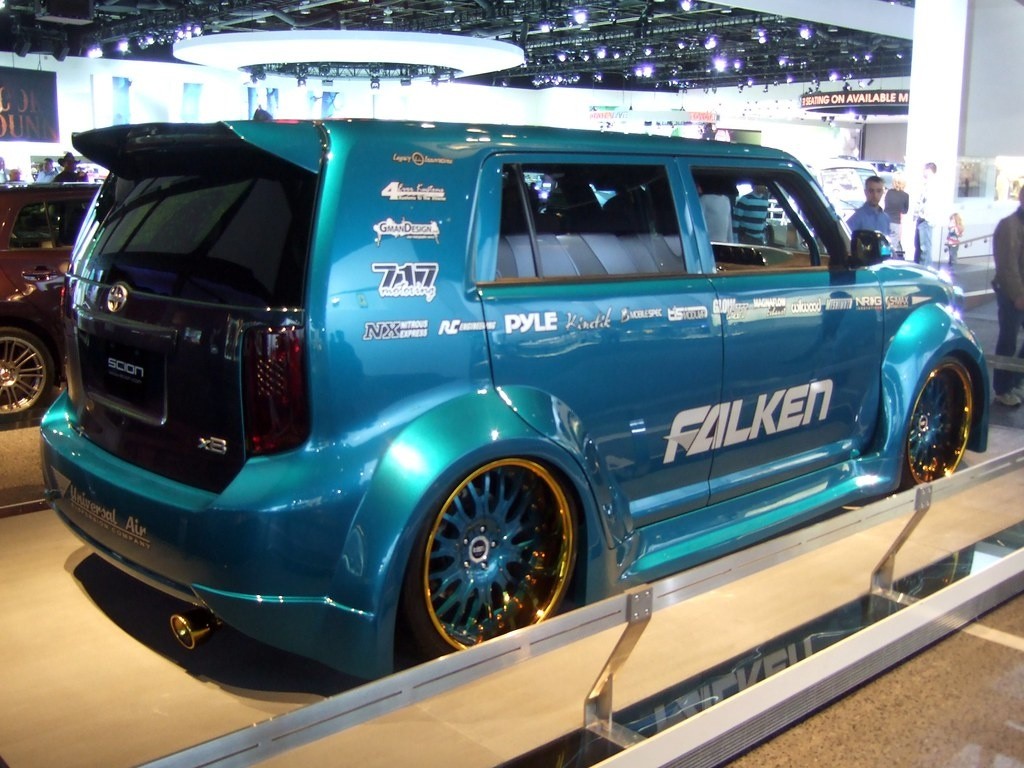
[810,163,886,218]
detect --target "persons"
[733,184,769,245]
[699,179,733,244]
[884,172,909,250]
[53,152,89,182]
[945,213,963,268]
[993,184,1024,405]
[912,162,948,266]
[36,158,58,183]
[846,175,890,239]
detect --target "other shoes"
[995,386,1024,406]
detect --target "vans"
[36,118,996,680]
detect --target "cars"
[0,180,104,430]
